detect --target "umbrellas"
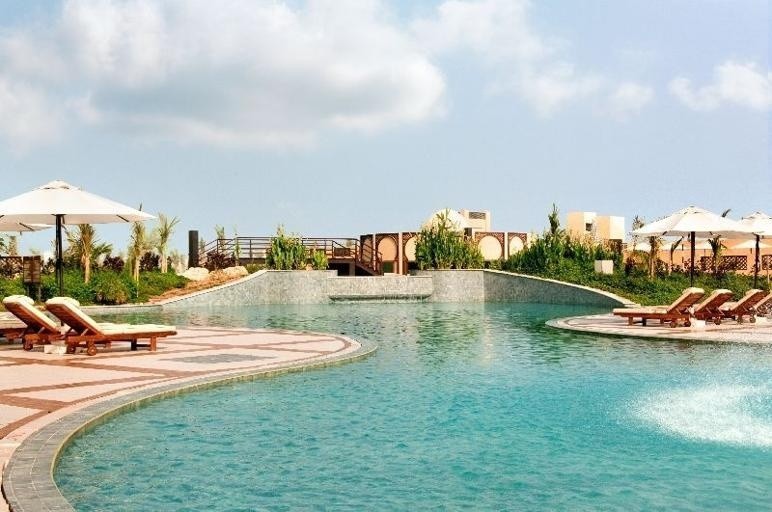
[0,178,159,327]
[628,203,765,289]
[731,209,772,290]
[0,222,52,233]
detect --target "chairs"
[718,288,766,322]
[750,291,772,318]
[45,297,178,357]
[0,294,131,351]
[0,320,28,344]
[611,285,706,327]
[691,288,732,325]
